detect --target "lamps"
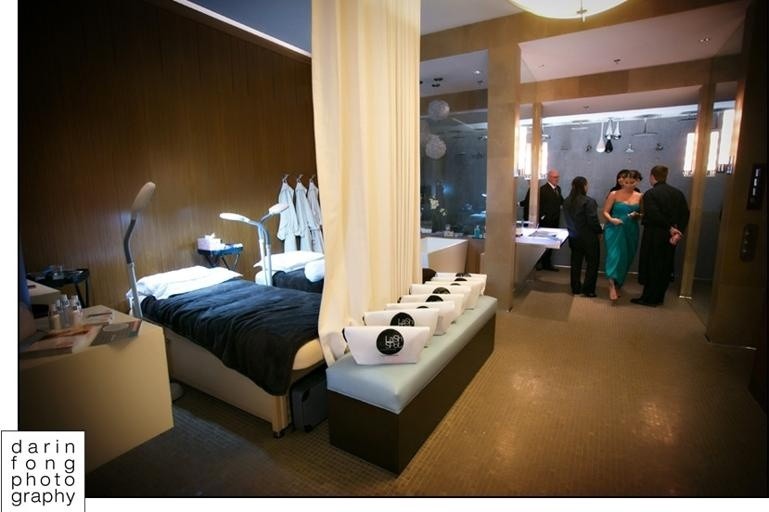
[123,179,183,403]
[595,116,623,154]
[258,202,290,287]
[683,107,736,180]
[219,210,273,289]
[428,99,450,123]
[537,141,549,181]
[423,133,446,159]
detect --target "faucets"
[532,214,549,231]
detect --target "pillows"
[136,264,244,300]
[252,250,324,274]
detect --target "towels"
[343,269,488,364]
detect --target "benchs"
[326,296,497,478]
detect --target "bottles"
[48,293,84,330]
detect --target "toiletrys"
[474,223,481,238]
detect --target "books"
[81,304,113,324]
[19,326,97,353]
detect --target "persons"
[601,171,643,303]
[516,178,530,226]
[561,174,602,298]
[608,167,641,198]
[535,170,564,274]
[629,164,690,309]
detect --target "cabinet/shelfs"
[18,302,175,480]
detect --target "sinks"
[525,230,565,241]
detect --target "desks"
[18,243,63,311]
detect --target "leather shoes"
[572,290,597,297]
[631,298,664,306]
[609,287,620,300]
[537,267,559,271]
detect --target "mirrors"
[515,120,533,231]
[419,108,487,241]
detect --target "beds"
[125,276,330,441]
[254,267,437,295]
